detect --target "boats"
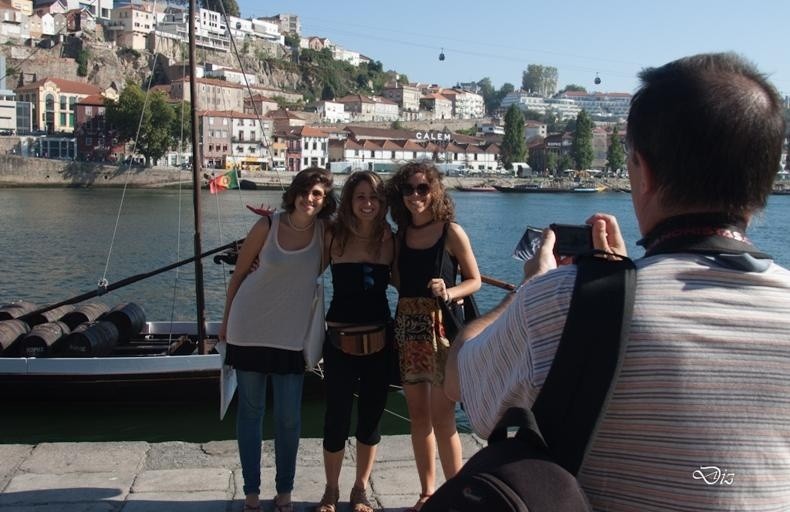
[453,175,632,194]
[769,183,790,195]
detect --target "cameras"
[549,222,595,257]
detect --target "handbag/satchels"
[392,296,450,388]
[301,282,327,373]
[327,320,391,358]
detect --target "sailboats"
[0,1,406,402]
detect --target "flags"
[206,169,240,195]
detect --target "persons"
[216,168,393,510]
[243,169,465,511]
[383,159,482,512]
[442,50,789,509]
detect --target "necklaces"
[352,226,373,244]
[285,213,315,232]
[408,215,437,230]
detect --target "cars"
[33,130,48,135]
[272,165,286,171]
[123,158,144,167]
[0,129,16,136]
[176,163,186,170]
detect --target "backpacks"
[417,404,601,512]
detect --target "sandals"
[241,497,264,512]
[404,494,434,512]
[349,484,374,512]
[315,484,340,512]
[273,495,295,512]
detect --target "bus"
[240,159,270,172]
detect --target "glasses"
[399,183,432,197]
[298,190,327,200]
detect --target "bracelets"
[444,288,451,305]
[511,284,525,295]
